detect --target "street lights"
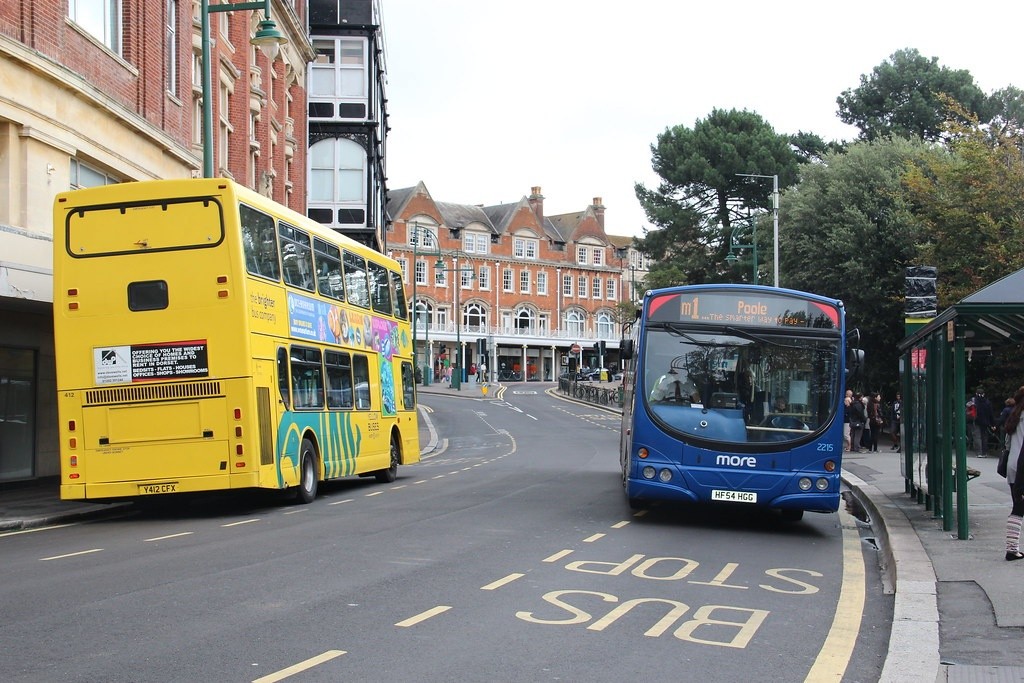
[200,0,289,180]
[442,247,479,390]
[52,177,421,504]
[724,216,758,286]
[412,220,446,378]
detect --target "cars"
[582,368,613,382]
[615,370,624,380]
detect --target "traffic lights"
[593,342,599,354]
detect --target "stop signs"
[572,345,580,354]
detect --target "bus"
[159,210,163,213]
[618,284,846,522]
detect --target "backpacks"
[975,397,990,426]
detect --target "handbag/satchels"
[997,446,1009,478]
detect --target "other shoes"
[1006,552,1024,561]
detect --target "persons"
[997,388,1024,560]
[439,364,455,383]
[469,361,489,383]
[843,386,1016,459]
[575,364,581,378]
[649,358,702,404]
[739,370,762,402]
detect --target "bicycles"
[600,386,619,405]
[575,382,597,398]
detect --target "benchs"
[952,464,981,482]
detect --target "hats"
[976,389,985,394]
